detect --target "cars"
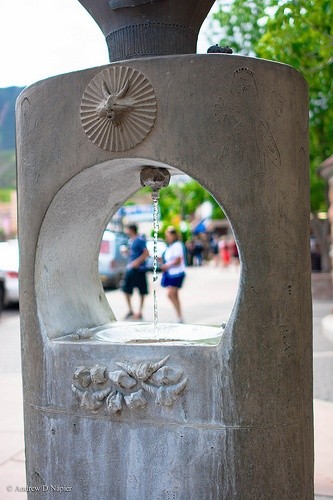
[1,232,167,309]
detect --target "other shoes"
[123,312,142,320]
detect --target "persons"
[115,219,148,318]
[184,230,239,272]
[309,230,321,271]
[161,226,188,324]
